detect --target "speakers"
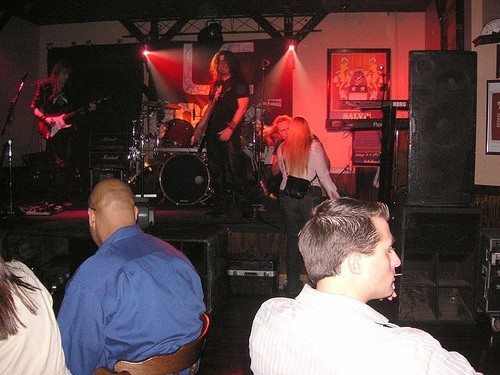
[396,49,484,324]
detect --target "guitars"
[38,95,113,140]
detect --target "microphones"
[262,58,270,66]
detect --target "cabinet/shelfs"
[396,204,484,327]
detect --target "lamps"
[196,19,224,50]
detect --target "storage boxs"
[226,252,280,297]
[477,228,500,331]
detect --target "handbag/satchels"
[283,176,310,199]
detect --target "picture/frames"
[327,47,392,129]
[485,79,500,155]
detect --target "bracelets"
[228,119,236,132]
[41,114,47,121]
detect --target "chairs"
[94,312,210,375]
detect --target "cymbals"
[254,105,284,110]
[162,105,181,110]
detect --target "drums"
[160,118,194,148]
[153,152,214,206]
[240,122,253,145]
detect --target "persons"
[29,61,98,208]
[141,108,169,208]
[190,50,254,219]
[258,110,333,233]
[0,251,73,375]
[55,179,207,375]
[249,196,484,375]
[276,116,342,301]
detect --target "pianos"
[326,118,409,204]
[339,100,409,110]
